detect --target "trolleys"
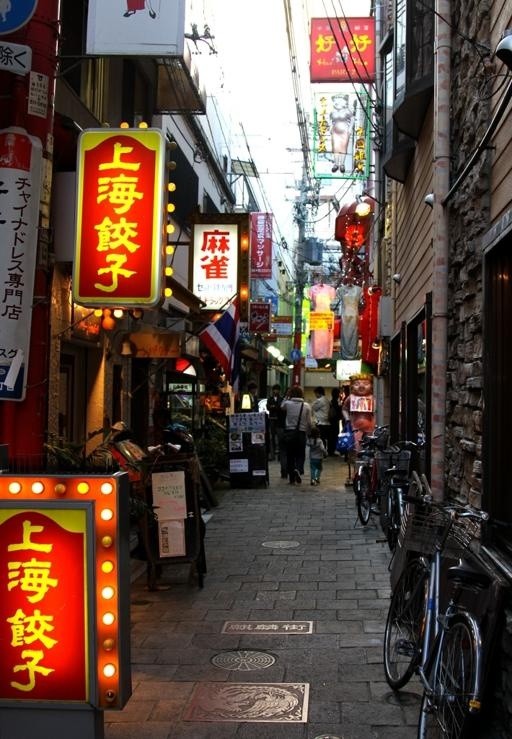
[139,452,201,592]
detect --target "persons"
[123,1,156,20]
[328,93,355,172]
[306,279,336,358]
[359,284,383,364]
[244,381,258,413]
[337,282,363,360]
[341,372,376,451]
[259,384,345,486]
[416,388,426,433]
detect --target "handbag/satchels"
[281,430,299,449]
[335,422,356,452]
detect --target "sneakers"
[289,468,320,486]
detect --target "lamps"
[355,191,390,217]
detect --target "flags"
[200,297,246,386]
